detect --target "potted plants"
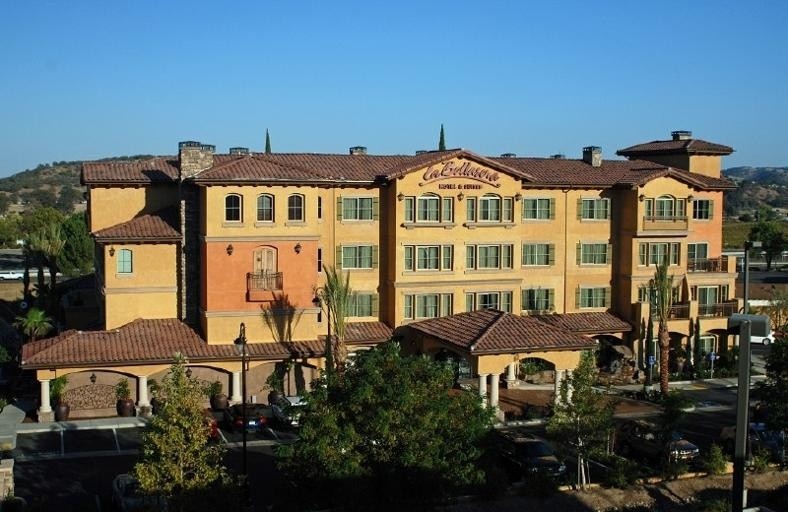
[209,380,227,408]
[54,376,69,421]
[115,376,133,416]
[267,375,284,405]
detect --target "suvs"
[272,396,312,431]
[0,270,24,281]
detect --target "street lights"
[313,285,340,407]
[234,321,248,479]
[726,313,770,512]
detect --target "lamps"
[639,194,645,200]
[88,374,96,383]
[226,244,233,255]
[515,192,521,201]
[186,367,192,378]
[688,194,693,202]
[294,242,303,254]
[398,191,404,201]
[457,191,463,201]
[107,245,114,257]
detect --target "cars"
[617,417,701,468]
[223,403,265,434]
[501,435,567,484]
[200,409,217,438]
[750,329,777,346]
[721,418,787,464]
